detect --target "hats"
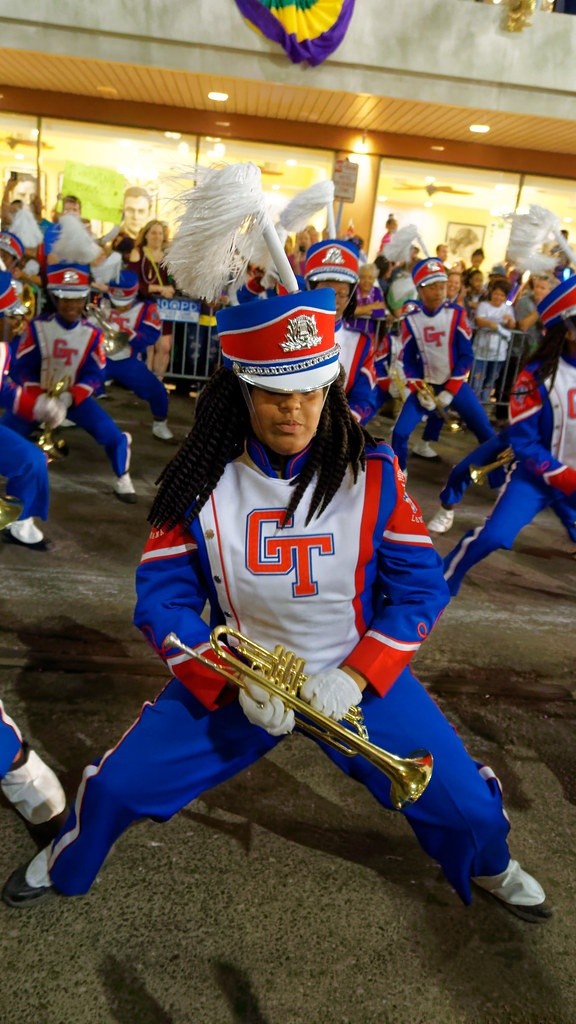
[280,179,362,283]
[0,228,142,315]
[164,164,342,393]
[383,223,449,290]
[508,206,576,329]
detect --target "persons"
[426,274,576,597]
[0,286,557,923]
[10,173,39,208]
[0,700,72,851]
[0,196,178,551]
[373,221,509,491]
[170,225,576,430]
[94,188,152,249]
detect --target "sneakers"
[427,506,453,534]
[6,518,52,550]
[3,848,55,909]
[151,418,178,445]
[412,440,442,463]
[113,471,139,503]
[3,741,69,848]
[472,857,556,923]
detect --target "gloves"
[301,665,362,720]
[239,676,294,738]
[37,390,69,430]
[416,386,451,412]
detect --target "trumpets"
[467,447,516,486]
[84,302,131,357]
[33,372,69,451]
[162,623,436,812]
[381,359,406,402]
[410,374,465,433]
